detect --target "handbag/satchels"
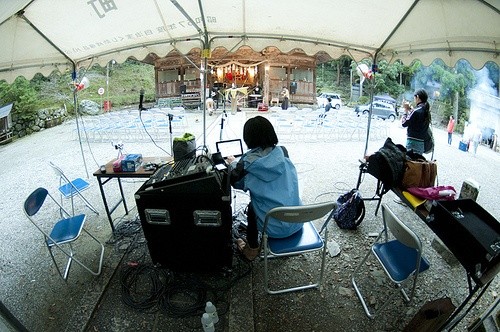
[424,127,434,154]
[366,137,437,188]
[406,185,456,201]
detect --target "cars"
[355,101,395,123]
[316,91,340,109]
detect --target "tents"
[0,0,499,182]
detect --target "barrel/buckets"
[104,101,111,110]
[459,141,467,151]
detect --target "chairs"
[22,187,105,280]
[66,107,187,144]
[247,105,382,144]
[48,161,99,219]
[257,201,338,293]
[351,203,430,320]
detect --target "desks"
[93,156,173,233]
[355,161,499,332]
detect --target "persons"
[227,116,303,261]
[281,87,290,110]
[210,80,219,111]
[319,98,331,125]
[473,128,482,154]
[447,115,454,146]
[402,88,431,156]
[254,81,261,95]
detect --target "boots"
[237,238,260,261]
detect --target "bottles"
[202,301,219,332]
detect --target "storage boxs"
[459,141,468,151]
[122,153,142,172]
[434,198,500,280]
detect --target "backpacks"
[333,189,365,229]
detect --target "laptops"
[217,138,244,164]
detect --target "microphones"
[139,89,144,111]
